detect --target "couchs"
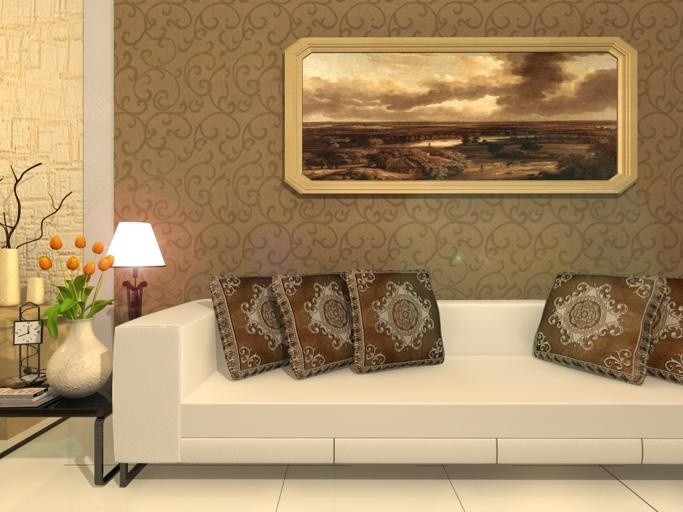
[112,298,683,487]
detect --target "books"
[0,386,48,398]
[0,392,62,408]
[0,390,53,403]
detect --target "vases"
[0,249,20,308]
[27,278,45,305]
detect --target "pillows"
[209,269,445,381]
[532,272,683,386]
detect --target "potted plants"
[39,236,115,400]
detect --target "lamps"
[106,221,166,321]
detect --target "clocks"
[13,301,43,386]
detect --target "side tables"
[0,373,120,486]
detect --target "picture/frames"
[284,38,640,196]
